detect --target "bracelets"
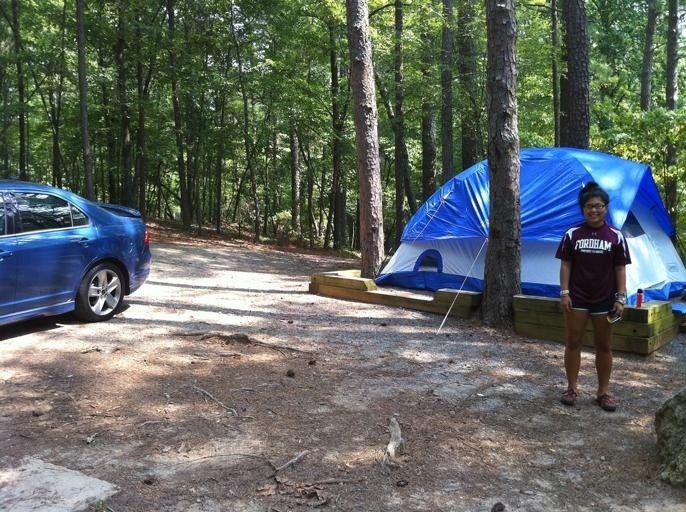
[558,289,570,297]
[614,292,627,306]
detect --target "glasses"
[583,203,606,210]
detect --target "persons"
[553,180,632,413]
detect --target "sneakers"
[560,389,577,405]
[598,394,616,411]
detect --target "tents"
[373,146,686,315]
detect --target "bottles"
[636,288,644,308]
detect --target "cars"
[0,178,152,338]
